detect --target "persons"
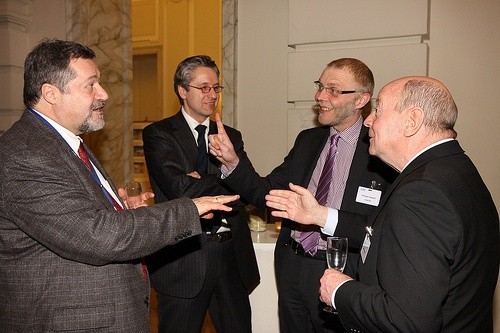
[143,54,265,333]
[265,77,500,333]
[208,57,399,332]
[0,36,241,333]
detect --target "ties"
[298,133,342,258]
[77,142,147,285]
[194,125,208,177]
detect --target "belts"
[205,232,232,242]
[290,239,328,260]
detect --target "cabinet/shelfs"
[131,0,223,333]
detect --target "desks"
[247,230,280,333]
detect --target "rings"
[216,198,219,203]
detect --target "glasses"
[185,84,225,94]
[313,81,356,96]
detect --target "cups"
[246,204,267,231]
[125,182,142,210]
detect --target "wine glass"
[322,236,348,314]
